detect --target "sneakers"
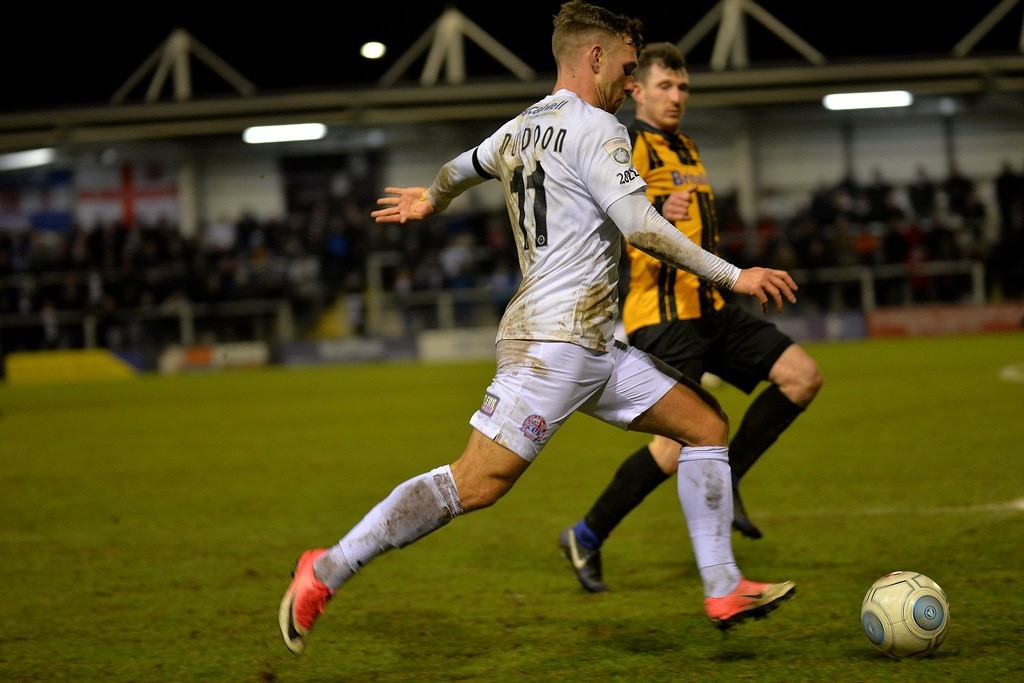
[705,578,797,631]
[561,527,606,592]
[729,486,761,538]
[279,548,335,656]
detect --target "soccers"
[860,571,951,659]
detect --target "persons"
[277,2,799,651]
[560,42,821,594]
[2,158,1024,376]
